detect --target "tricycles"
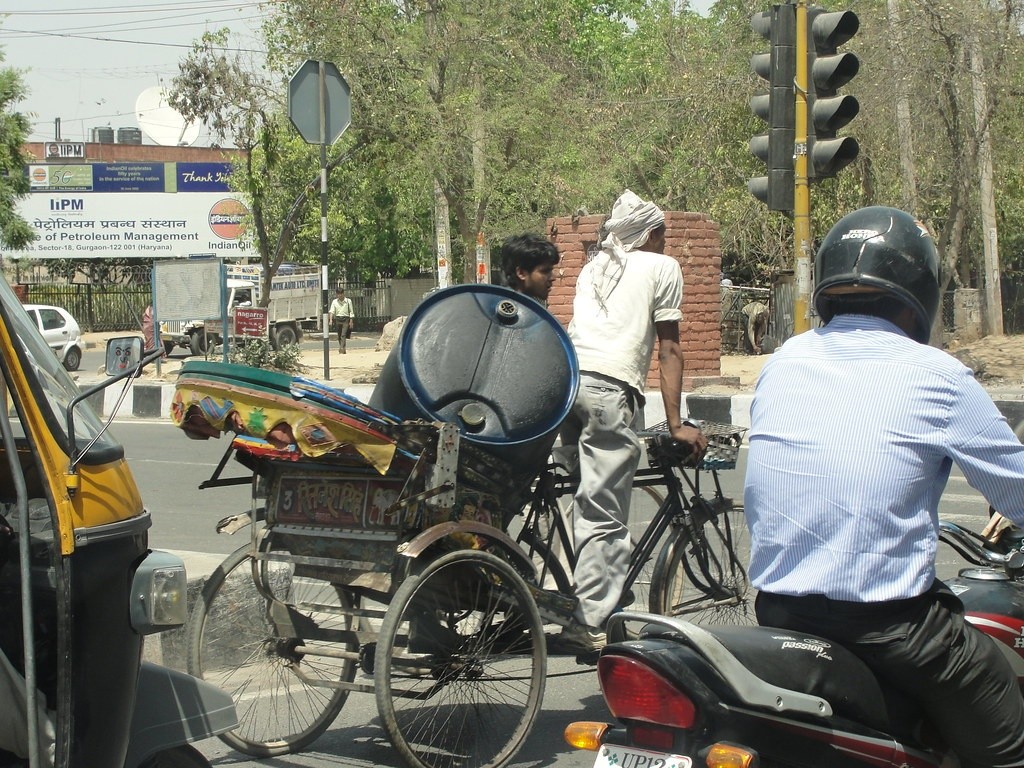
[175,420,770,766]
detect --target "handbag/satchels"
[345,325,352,338]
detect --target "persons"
[328,288,354,354]
[742,301,769,357]
[560,189,708,666]
[743,206,1024,768]
[721,274,733,286]
[142,299,168,363]
[458,232,561,625]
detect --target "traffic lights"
[797,7,860,183]
[747,4,799,212]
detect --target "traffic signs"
[233,306,270,343]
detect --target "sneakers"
[551,619,607,656]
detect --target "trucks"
[0,274,191,768]
[160,261,323,360]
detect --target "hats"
[593,189,666,306]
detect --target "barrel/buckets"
[368,284,583,534]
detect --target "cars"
[21,304,85,373]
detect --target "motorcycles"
[565,515,1024,768]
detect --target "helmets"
[812,205,940,346]
